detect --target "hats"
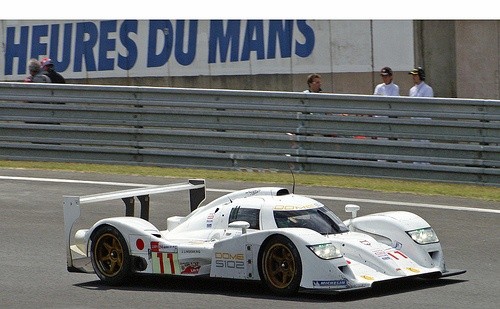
[379,66,393,75]
[28,58,42,70]
[408,66,424,74]
[40,57,54,68]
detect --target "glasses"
[313,81,320,84]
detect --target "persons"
[408,67,433,165]
[24,56,66,145]
[373,66,402,163]
[287,211,310,228]
[297,74,334,137]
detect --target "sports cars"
[61,177,469,297]
